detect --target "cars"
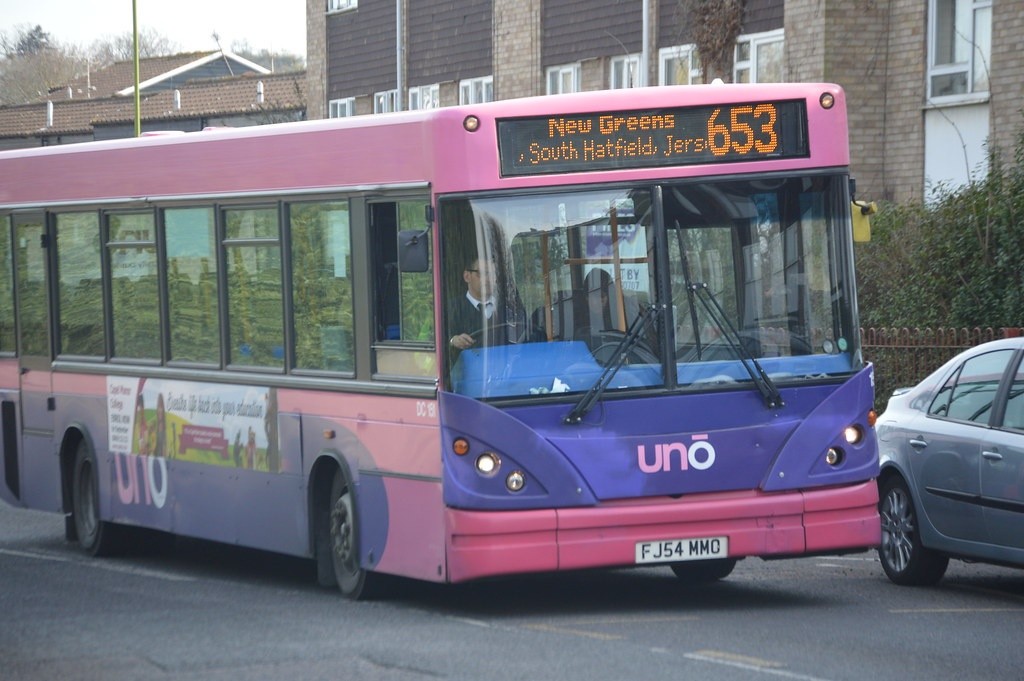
[874,336,1024,583]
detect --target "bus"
[0,82,886,601]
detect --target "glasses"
[467,269,489,278]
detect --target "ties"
[478,303,491,312]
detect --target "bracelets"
[450,337,454,344]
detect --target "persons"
[565,268,658,352]
[447,252,532,366]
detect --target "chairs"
[319,325,355,372]
